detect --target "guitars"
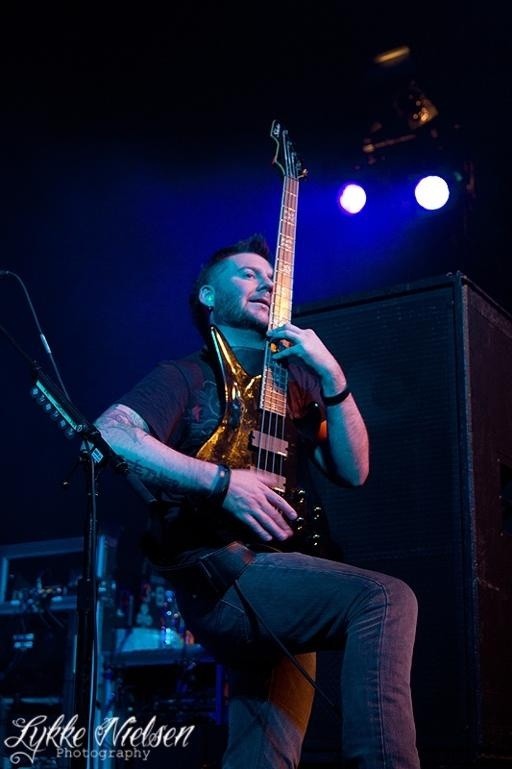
[174,120,333,559]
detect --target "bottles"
[158,590,181,647]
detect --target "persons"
[91,231,423,769]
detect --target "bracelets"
[317,382,355,407]
[208,461,231,514]
